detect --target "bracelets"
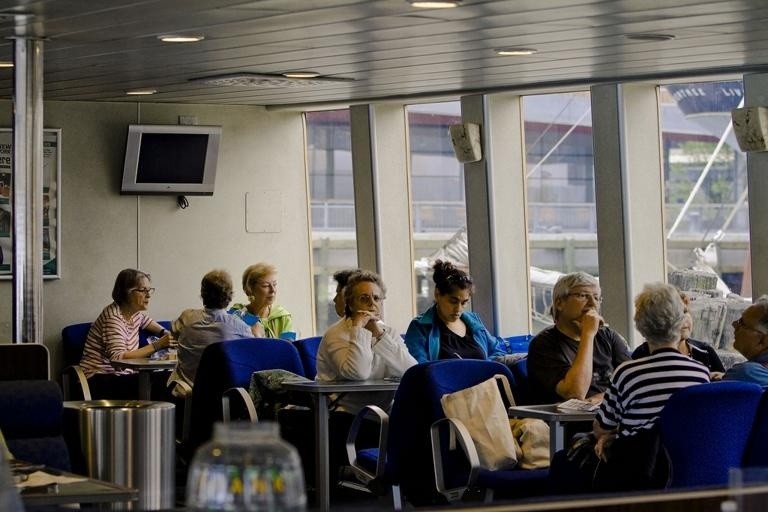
[159,328,165,338]
[152,341,160,352]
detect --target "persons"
[402,258,507,367]
[708,295,768,391]
[72,265,179,400]
[546,281,713,495]
[527,271,634,405]
[227,262,297,344]
[335,269,360,318]
[156,268,258,405]
[302,272,423,456]
[630,288,727,373]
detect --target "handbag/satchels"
[510,418,551,469]
[440,374,522,472]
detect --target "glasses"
[444,275,471,283]
[135,287,155,296]
[738,316,767,336]
[355,296,382,303]
[570,291,603,303]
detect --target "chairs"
[335,359,442,510]
[499,335,534,354]
[292,336,322,381]
[188,338,316,482]
[415,359,550,509]
[0,379,72,473]
[138,321,172,358]
[61,322,103,401]
[509,358,527,395]
[638,381,762,493]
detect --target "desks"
[9,464,140,506]
[110,358,177,401]
[278,378,401,511]
[508,402,599,467]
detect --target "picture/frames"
[0,126,62,280]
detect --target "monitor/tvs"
[119,124,223,196]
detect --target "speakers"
[730,106,768,155]
[447,123,482,166]
